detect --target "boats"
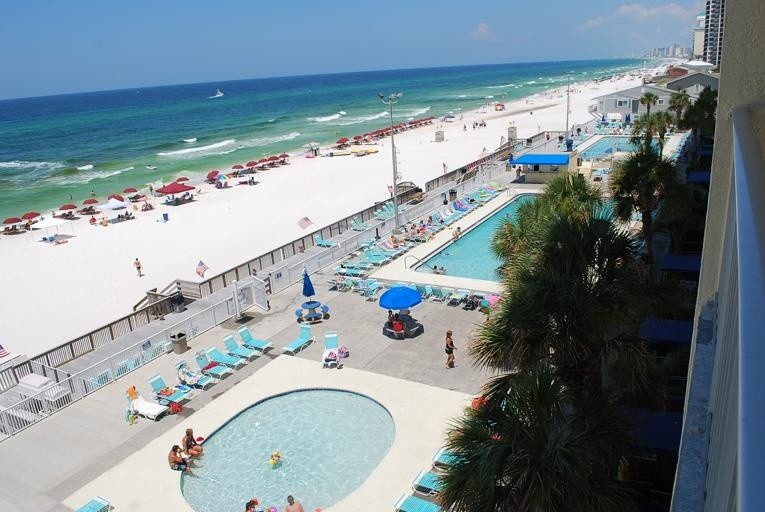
[147,165,157,170]
[216,88,223,96]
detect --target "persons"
[3,116,434,226]
[244,503,252,512]
[392,313,404,337]
[250,500,255,512]
[182,429,203,457]
[444,331,458,369]
[391,216,462,246]
[515,166,522,176]
[285,495,303,512]
[388,310,395,324]
[168,445,203,478]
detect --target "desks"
[397,314,413,323]
[301,301,320,310]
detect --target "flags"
[0,345,9,358]
[196,261,208,279]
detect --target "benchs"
[383,321,423,340]
[295,305,328,323]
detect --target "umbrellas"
[379,286,422,310]
[303,270,315,301]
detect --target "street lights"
[378,89,407,234]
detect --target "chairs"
[90,322,272,421]
[560,120,671,150]
[410,470,444,497]
[314,235,335,248]
[74,495,111,512]
[393,491,442,512]
[669,130,692,163]
[321,329,339,368]
[281,325,314,356]
[430,447,469,473]
[331,181,504,316]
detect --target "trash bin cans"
[163,214,168,221]
[449,189,457,202]
[172,295,185,313]
[460,167,467,177]
[170,332,188,354]
[567,140,574,151]
